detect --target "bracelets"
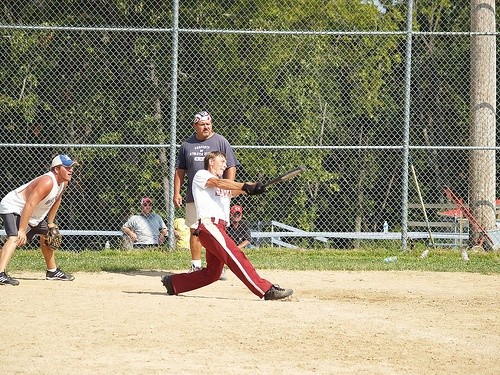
[47,222,54,224]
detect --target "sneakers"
[0,269,20,286]
[46,267,75,281]
[264,284,294,300]
[161,275,174,296]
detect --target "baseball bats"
[250,165,304,197]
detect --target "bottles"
[384,256,397,262]
[105,241,111,250]
[419,250,429,259]
[383,221,388,233]
[461,249,468,260]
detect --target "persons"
[173,217,191,250]
[225,205,251,250]
[161,150,294,301]
[121,198,167,249]
[173,111,240,280]
[0,154,78,287]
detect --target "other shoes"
[187,264,203,274]
[219,266,227,280]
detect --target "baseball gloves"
[44,222,63,251]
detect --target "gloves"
[242,183,266,195]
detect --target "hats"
[231,205,242,215]
[194,111,212,124]
[50,154,77,170]
[140,195,152,202]
